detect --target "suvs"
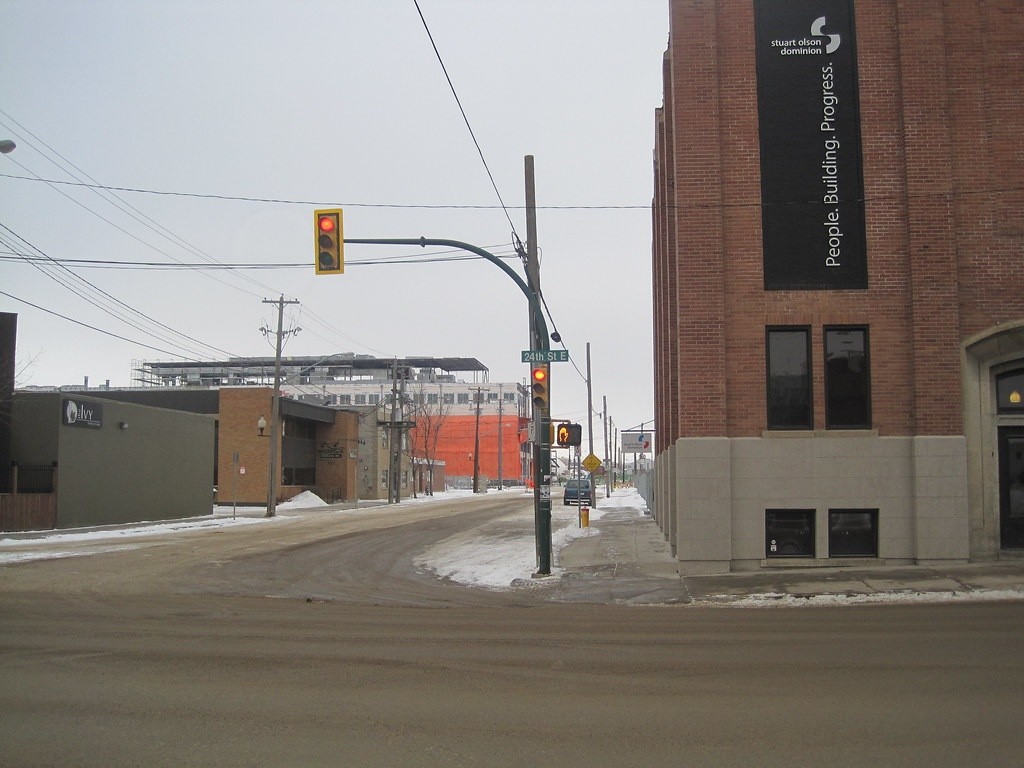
[563,479,592,506]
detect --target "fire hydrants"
[426,487,429,496]
[581,508,589,528]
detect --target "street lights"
[258,414,277,517]
[468,452,478,493]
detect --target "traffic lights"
[557,424,581,445]
[532,367,549,408]
[314,208,344,274]
